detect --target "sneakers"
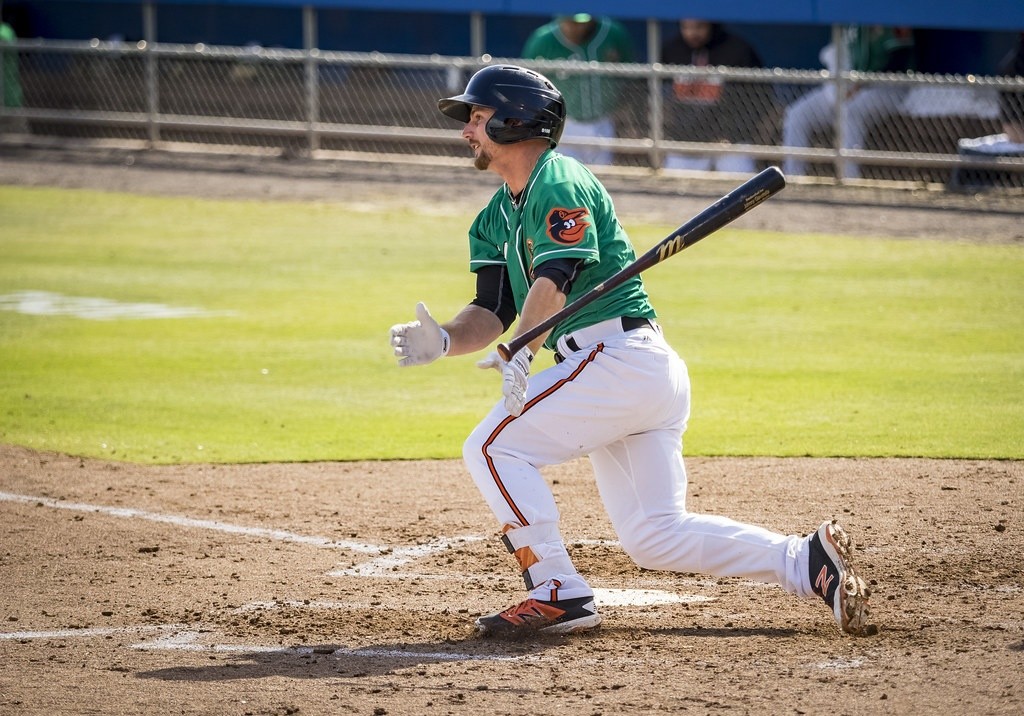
[473,595,602,638]
[808,519,871,633]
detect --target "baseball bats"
[495,164,789,364]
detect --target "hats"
[555,11,595,23]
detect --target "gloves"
[475,342,534,417]
[390,301,452,368]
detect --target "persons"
[0,22,29,149]
[522,14,635,162]
[781,24,917,177]
[389,65,869,634]
[629,18,777,173]
[949,47,1024,187]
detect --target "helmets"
[437,63,566,149]
